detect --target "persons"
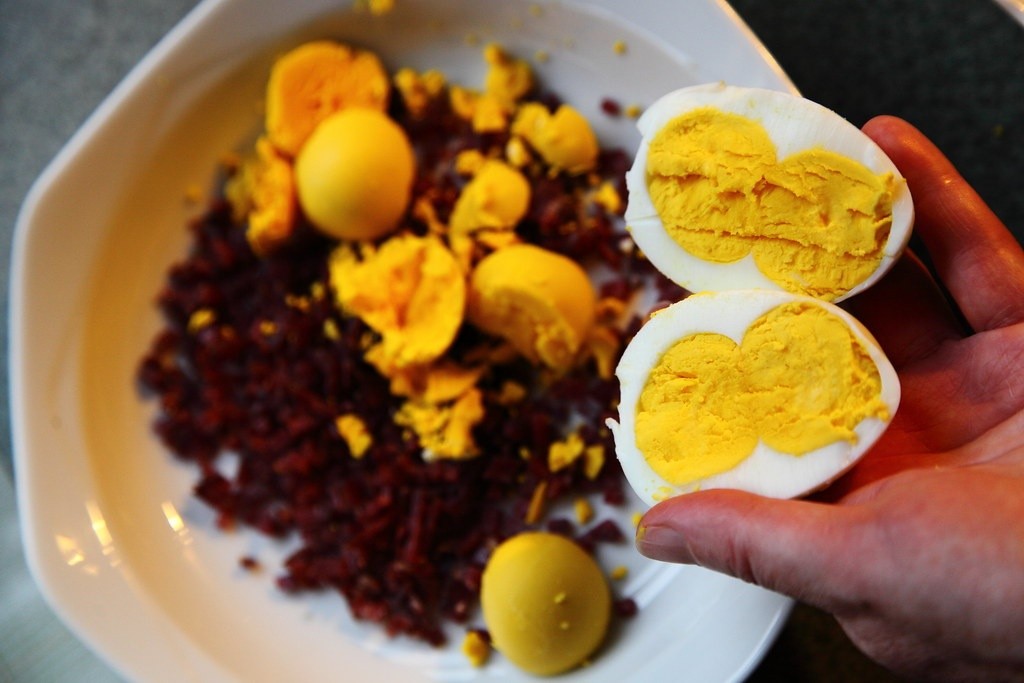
[635,117,1023,683]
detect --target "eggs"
[606,81,918,519]
[223,39,620,674]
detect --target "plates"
[8,0,805,683]
